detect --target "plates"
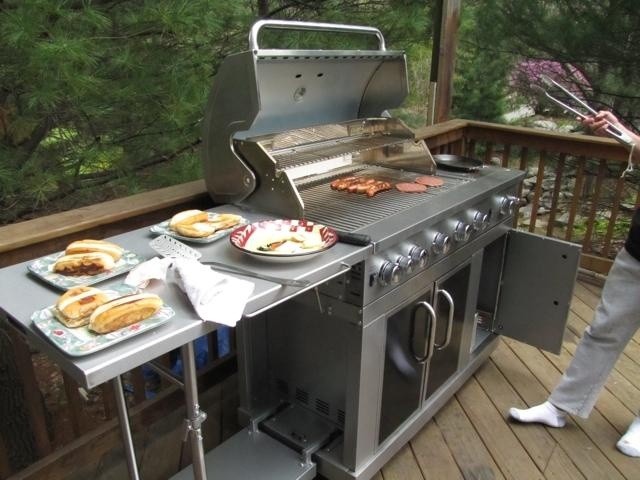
[432,153,484,173]
[27,244,147,294]
[31,281,175,359]
[229,219,338,264]
[149,210,249,246]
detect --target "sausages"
[331,175,392,197]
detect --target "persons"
[508,109,640,457]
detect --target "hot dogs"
[89,293,162,334]
[55,252,115,276]
[169,210,216,238]
[65,239,121,262]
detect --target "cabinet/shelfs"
[264,254,475,473]
[472,227,584,370]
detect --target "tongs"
[525,76,640,157]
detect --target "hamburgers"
[55,287,106,328]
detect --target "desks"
[0,203,375,480]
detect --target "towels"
[167,255,256,330]
